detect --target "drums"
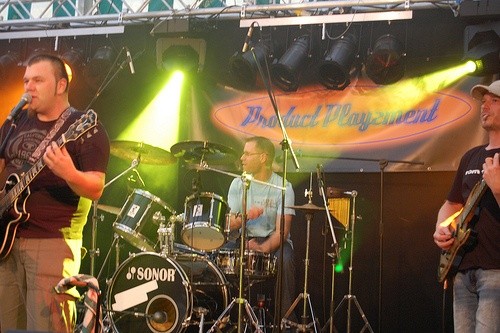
[216,249,277,280]
[106,251,228,333]
[180,191,229,252]
[111,189,176,253]
[162,242,208,256]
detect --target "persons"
[208,137,297,333]
[0,54,109,333]
[434,80,500,333]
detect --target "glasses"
[241,151,266,158]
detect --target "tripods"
[197,156,374,333]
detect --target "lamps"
[229,11,412,88]
[0,25,126,85]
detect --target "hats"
[470,79,500,98]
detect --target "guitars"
[0,109,99,262]
[437,175,488,284]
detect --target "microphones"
[317,164,323,196]
[3,93,32,127]
[126,48,135,74]
[242,23,255,52]
[132,169,144,186]
[192,178,200,195]
[275,152,300,162]
[153,312,168,323]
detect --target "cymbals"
[285,204,335,213]
[109,140,177,165]
[170,141,238,164]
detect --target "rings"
[481,170,484,174]
